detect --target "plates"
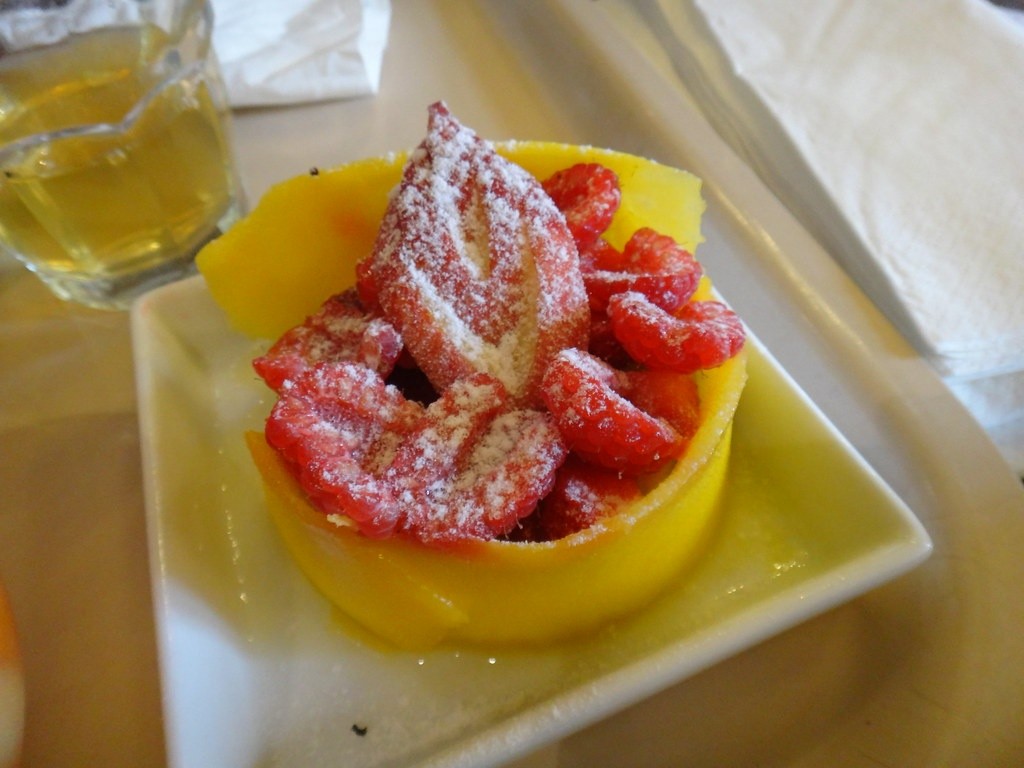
[127,267,933,766]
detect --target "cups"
[0,0,249,310]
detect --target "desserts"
[195,99,747,646]
